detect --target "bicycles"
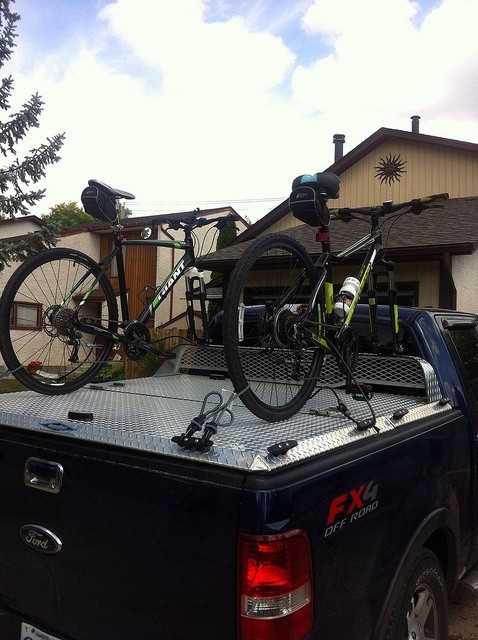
[222,173,448,424]
[0,179,241,396]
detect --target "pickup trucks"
[0,302,478,640]
